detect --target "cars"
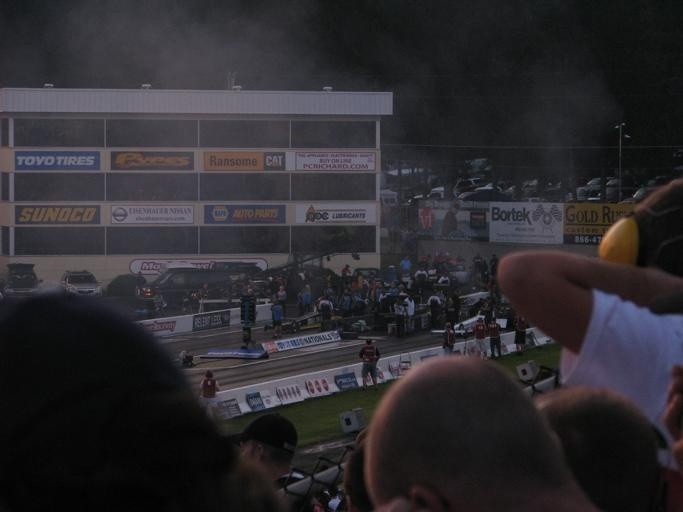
[2,261,43,298]
[56,270,103,299]
[381,172,664,203]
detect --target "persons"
[0,178,682,511]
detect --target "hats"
[228,413,297,454]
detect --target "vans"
[137,267,232,310]
